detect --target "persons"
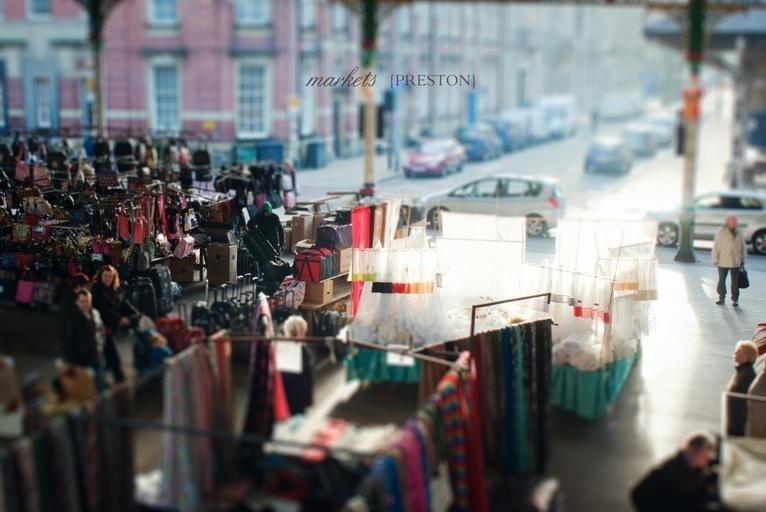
[247,202,285,257]
[627,430,719,511]
[91,265,139,383]
[62,289,101,372]
[275,316,315,416]
[710,214,745,308]
[724,339,758,436]
[58,274,89,333]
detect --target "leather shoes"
[731,299,740,306]
[715,298,725,305]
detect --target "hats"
[261,200,272,215]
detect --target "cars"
[402,138,465,175]
[646,191,765,255]
[581,136,634,173]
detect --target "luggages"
[150,264,173,316]
[176,273,259,335]
[137,277,158,321]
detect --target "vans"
[416,175,566,236]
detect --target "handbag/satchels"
[14,268,34,304]
[118,185,195,272]
[739,264,750,290]
[294,245,338,283]
[273,275,306,310]
[34,268,55,306]
[317,223,351,248]
[9,178,52,243]
[262,256,291,295]
[54,228,92,263]
[0,265,15,302]
